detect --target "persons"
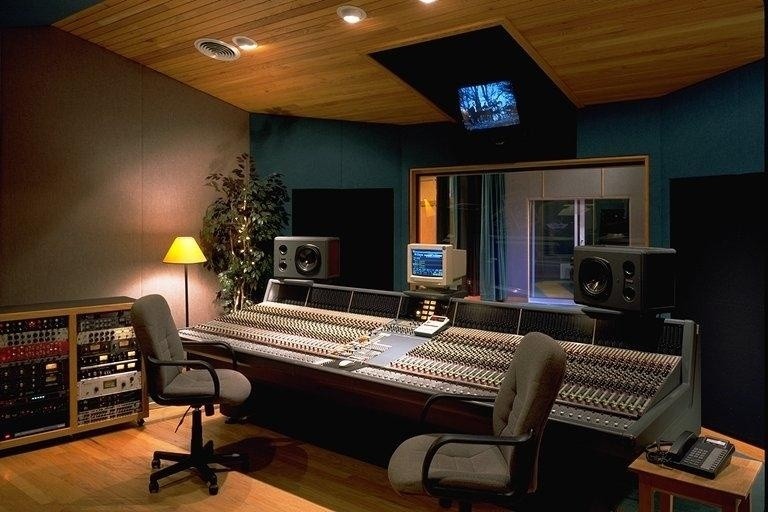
[460,97,498,124]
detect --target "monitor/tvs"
[405,243,469,289]
[456,77,522,132]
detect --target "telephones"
[662,430,736,479]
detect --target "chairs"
[129,292,253,493]
[384,329,568,511]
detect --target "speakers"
[573,245,680,312]
[274,236,340,278]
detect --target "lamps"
[161,235,208,326]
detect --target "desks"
[627,426,764,512]
